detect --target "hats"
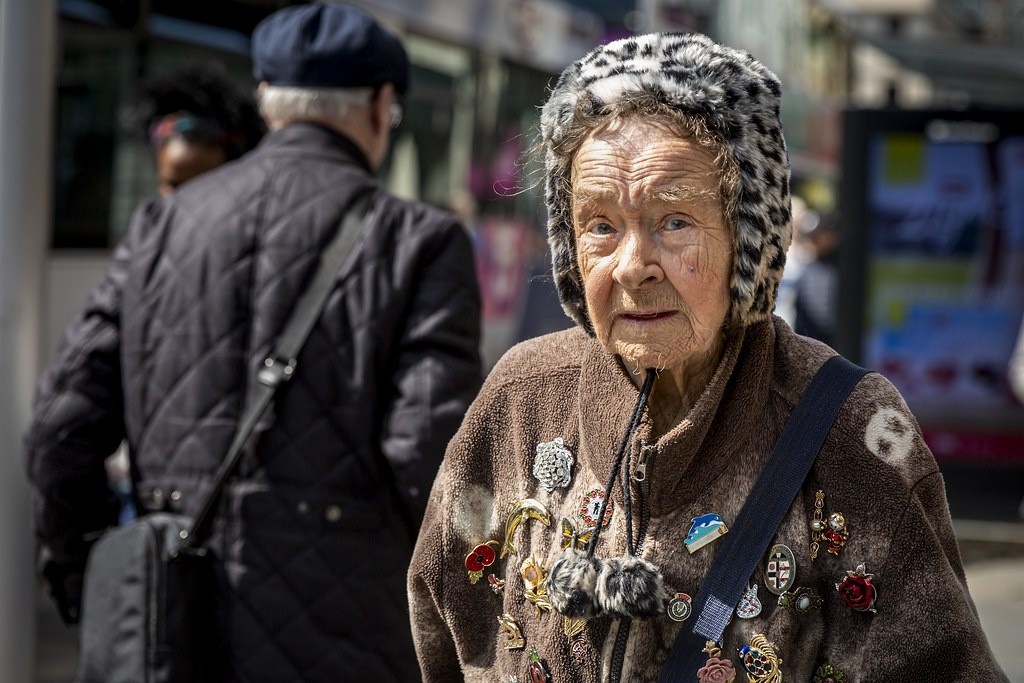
[536,33,794,337]
[252,3,410,100]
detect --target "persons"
[401,34,1009,683]
[25,2,488,683]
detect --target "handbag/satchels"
[82,517,227,683]
